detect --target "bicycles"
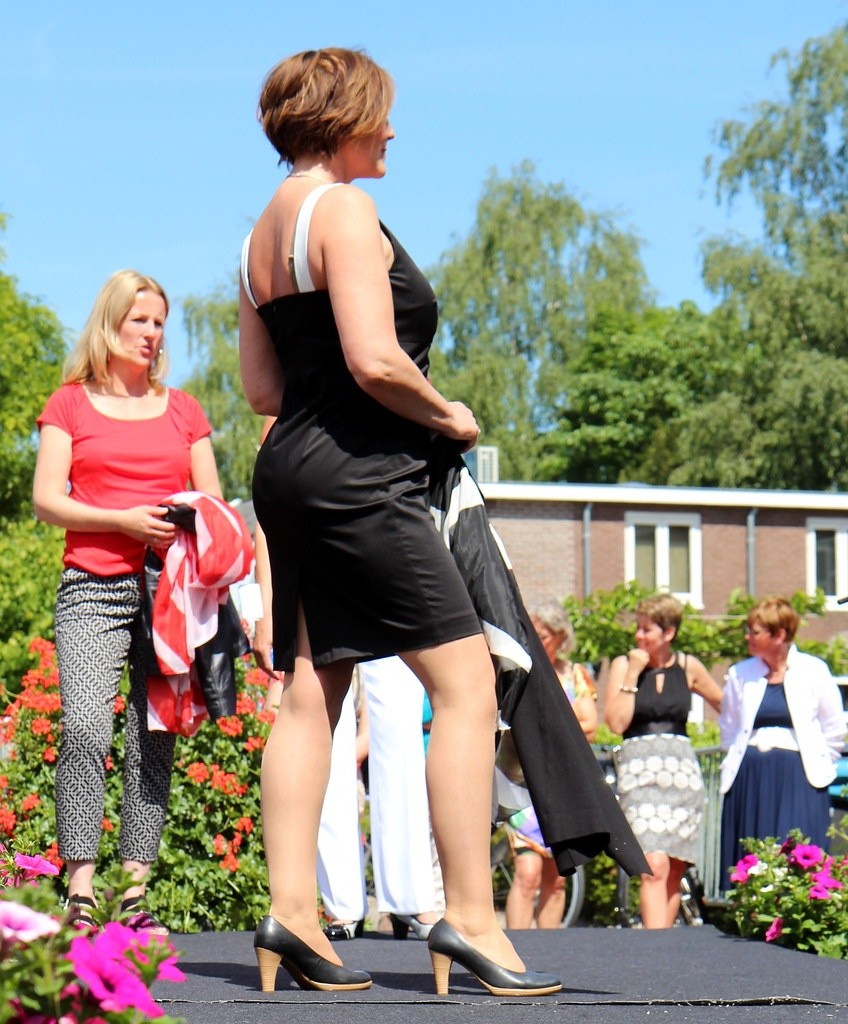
[362,834,582,928]
[612,860,710,929]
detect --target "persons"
[605,594,722,929]
[239,50,561,995]
[32,270,224,936]
[252,416,447,941]
[503,603,599,929]
[719,599,847,896]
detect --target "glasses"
[744,628,770,637]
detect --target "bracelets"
[621,685,638,694]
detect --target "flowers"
[721,829,848,960]
[0,860,187,1024]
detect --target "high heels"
[390,913,434,940]
[322,918,364,940]
[427,918,563,996]
[254,916,372,992]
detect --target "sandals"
[64,894,98,938]
[122,895,171,936]
